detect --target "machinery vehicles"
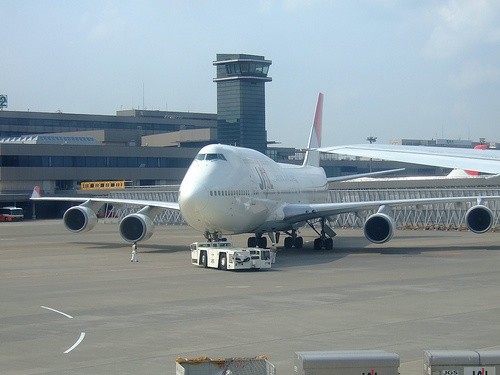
[191,242,271,270]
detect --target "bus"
[0,206,25,223]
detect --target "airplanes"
[29,92,500,249]
